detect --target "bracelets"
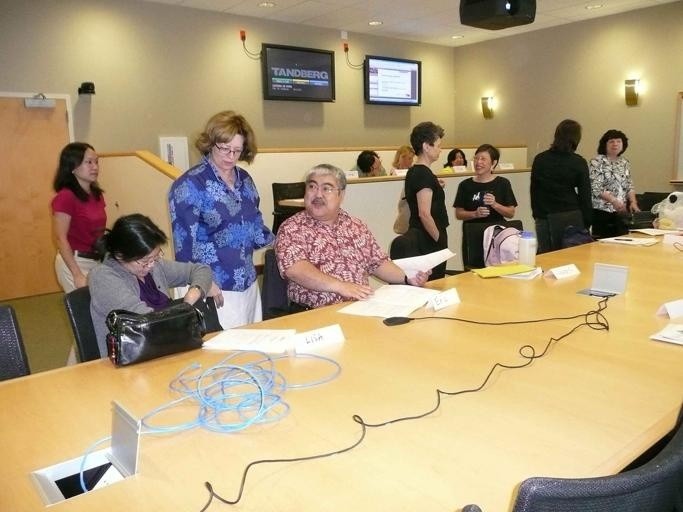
[405,276,409,285]
[188,285,204,294]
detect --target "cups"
[470,161,476,172]
[480,191,493,210]
[518,231,538,267]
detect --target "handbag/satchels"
[106,302,207,366]
[562,225,595,247]
[394,187,411,233]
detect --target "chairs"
[0,305,32,382]
[272,182,306,236]
[546,209,584,251]
[511,400,683,512]
[64,285,101,362]
[462,219,523,272]
[260,249,293,321]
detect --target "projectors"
[459,0,536,30]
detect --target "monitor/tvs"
[261,42,336,102]
[362,54,422,105]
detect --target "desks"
[0,231,683,512]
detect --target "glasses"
[128,250,166,269]
[214,143,241,156]
[307,184,340,194]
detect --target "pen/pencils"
[614,238,633,241]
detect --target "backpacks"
[483,224,521,266]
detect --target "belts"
[72,251,102,259]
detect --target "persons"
[439,148,467,175]
[530,119,592,254]
[405,121,450,282]
[453,144,518,272]
[588,129,641,213]
[87,214,212,359]
[274,164,433,313]
[350,150,387,177]
[390,145,414,175]
[168,110,276,330]
[51,143,108,366]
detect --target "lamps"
[481,97,495,120]
[625,80,639,106]
